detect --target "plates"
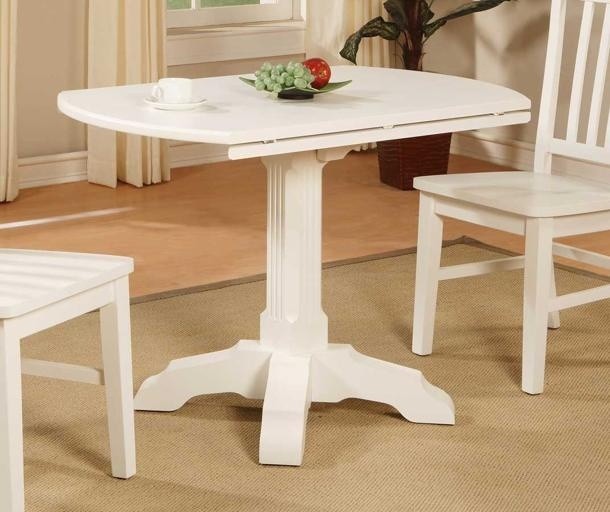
[237,76,352,100]
[145,92,208,111]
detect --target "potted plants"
[335,1,505,190]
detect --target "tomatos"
[304,57,330,89]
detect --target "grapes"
[254,60,315,92]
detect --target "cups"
[151,77,192,103]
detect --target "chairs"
[410,2,608,397]
[0,248,140,508]
